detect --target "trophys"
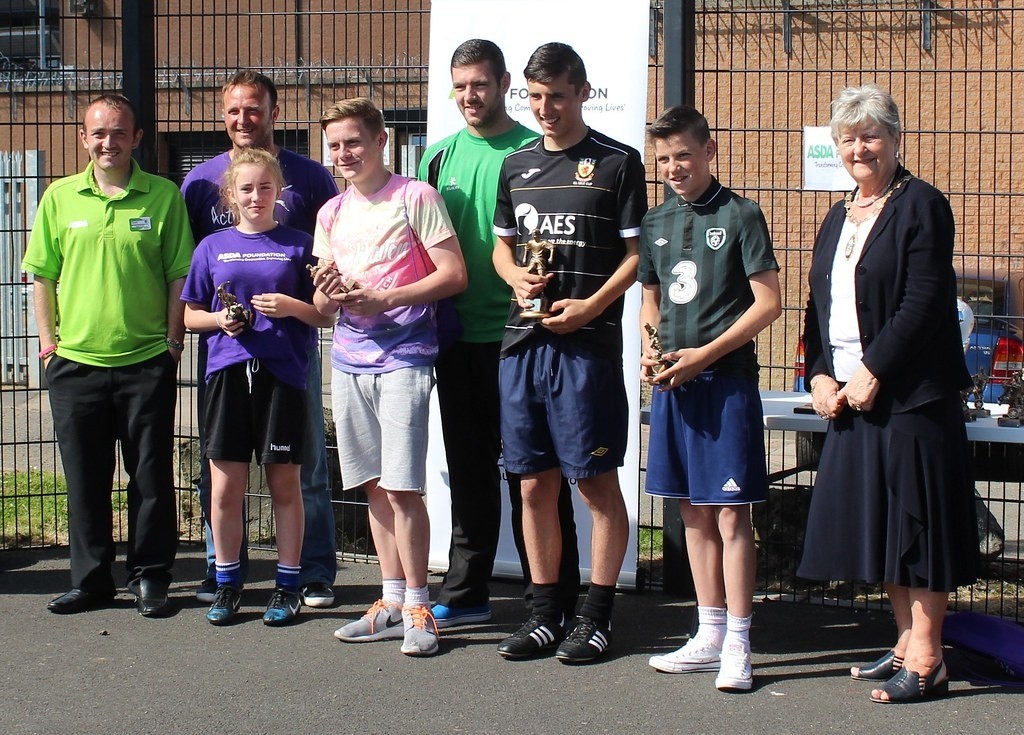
[960,367,1024,428]
[305,262,356,303]
[644,323,673,384]
[518,229,555,318]
[214,280,254,331]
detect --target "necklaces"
[843,166,917,258]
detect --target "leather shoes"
[136,578,168,616]
[47,588,116,610]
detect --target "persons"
[639,108,780,690]
[19,93,196,617]
[179,69,340,624]
[798,82,982,704]
[311,97,468,654]
[415,39,581,627]
[493,43,649,661]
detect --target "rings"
[856,406,863,412]
[820,415,829,420]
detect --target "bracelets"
[166,338,184,352]
[214,312,222,328]
[809,374,828,396]
[38,344,57,359]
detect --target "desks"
[640,387,1024,444]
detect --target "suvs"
[793,267,1024,472]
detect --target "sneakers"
[496,613,565,658]
[196,578,218,601]
[556,615,612,661]
[263,587,301,625]
[649,626,721,673]
[302,584,334,606]
[334,598,404,643]
[206,582,244,624]
[431,603,492,628]
[401,603,439,655]
[715,636,753,689]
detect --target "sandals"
[869,660,948,703]
[851,650,905,682]
